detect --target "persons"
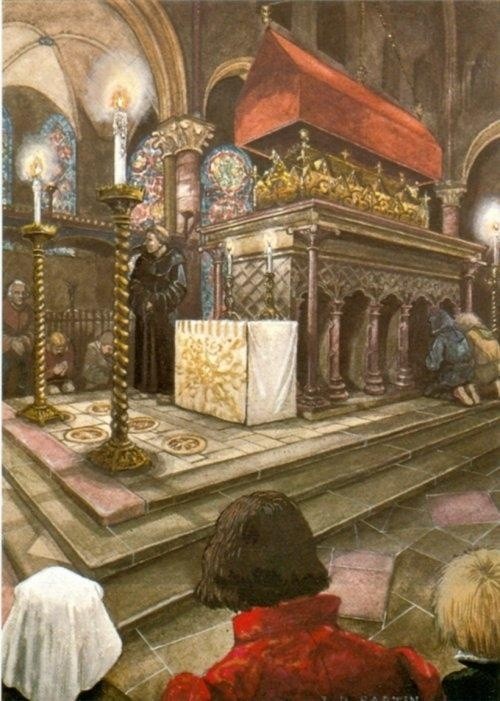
[127,224,188,405]
[454,312,499,398]
[44,332,76,394]
[80,331,115,390]
[2,277,36,398]
[430,548,500,701]
[160,490,444,701]
[2,565,124,700]
[423,310,480,407]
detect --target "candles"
[30,156,44,224]
[113,88,128,186]
[267,239,272,273]
[491,218,500,265]
[226,240,232,274]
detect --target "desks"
[175,318,299,427]
